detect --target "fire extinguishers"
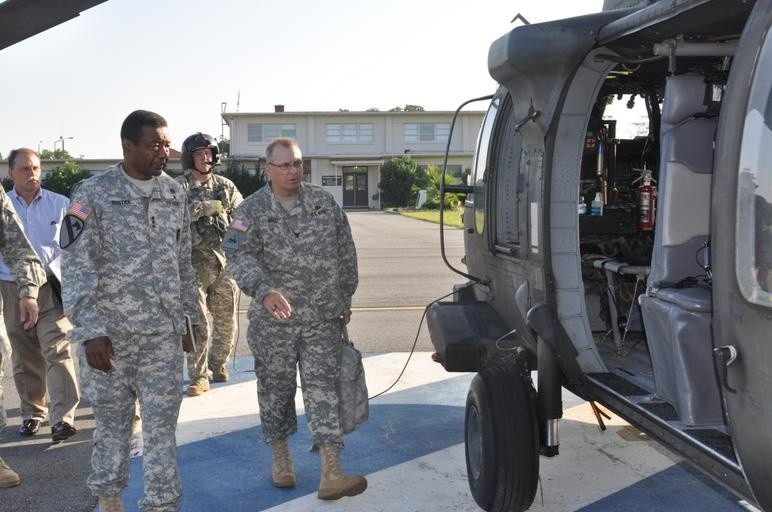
[631,167,657,228]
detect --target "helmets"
[180,133,221,170]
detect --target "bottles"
[579,196,587,214]
[594,133,608,178]
[590,192,604,217]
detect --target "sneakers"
[188,377,209,396]
[0,456,20,487]
[208,362,228,381]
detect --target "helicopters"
[0,0,771,511]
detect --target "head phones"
[181,145,219,169]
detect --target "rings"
[271,304,277,312]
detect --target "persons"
[174,130,245,396]
[220,136,368,499]
[0,183,42,490]
[59,110,204,512]
[0,148,79,439]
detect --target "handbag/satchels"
[339,344,368,433]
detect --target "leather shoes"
[51,421,75,440]
[20,421,39,434]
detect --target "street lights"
[38,139,42,154]
[54,136,73,153]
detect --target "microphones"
[206,161,216,165]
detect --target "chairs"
[637,67,732,436]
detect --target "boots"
[318,445,366,500]
[272,436,295,487]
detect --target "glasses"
[268,159,303,171]
